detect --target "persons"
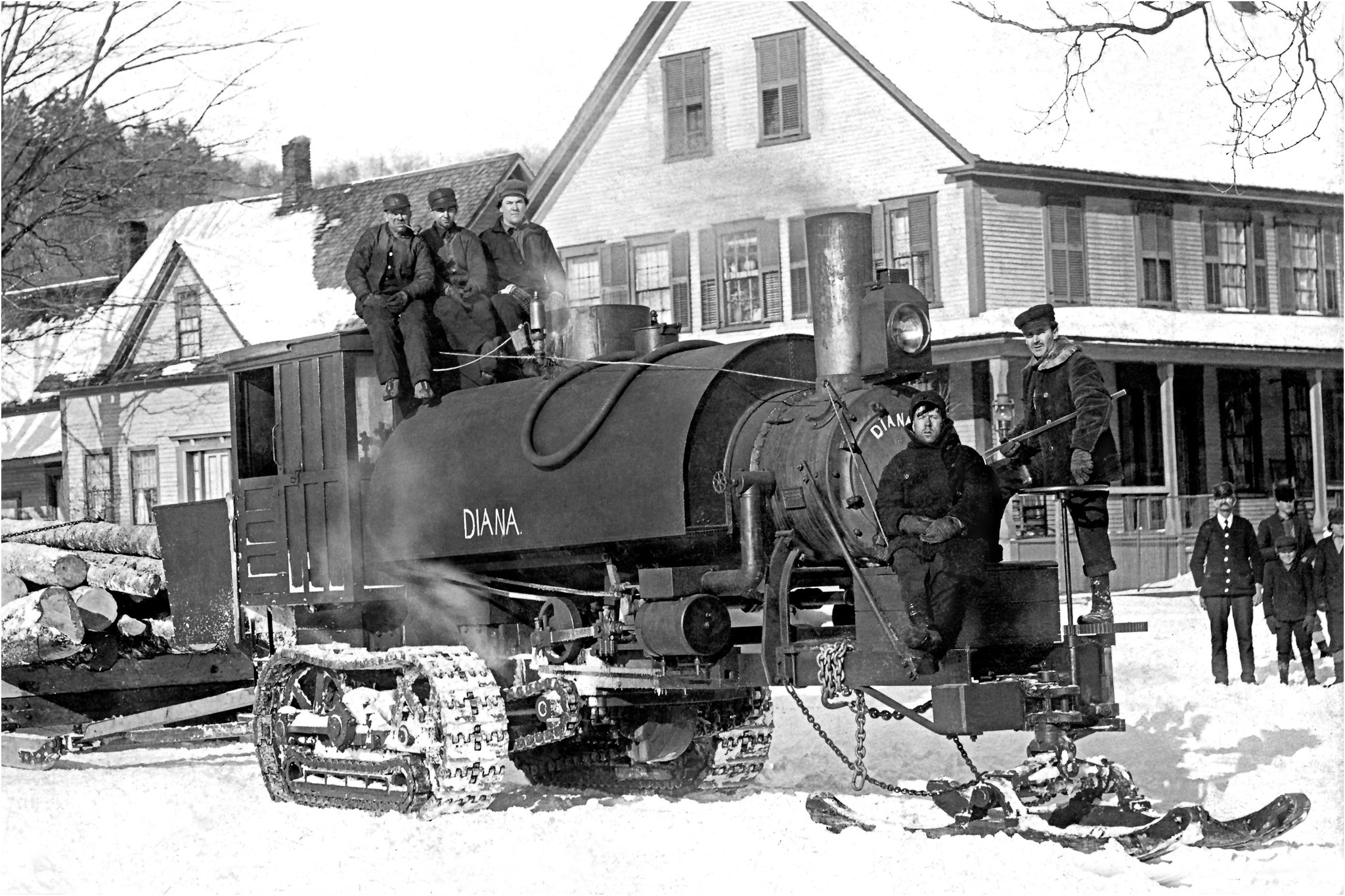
[986,304,1125,625]
[1257,486,1344,688]
[875,391,989,654]
[345,193,436,402]
[420,188,507,384]
[1190,480,1262,686]
[478,179,569,377]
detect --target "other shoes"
[414,382,435,398]
[522,361,560,378]
[460,343,508,390]
[383,378,403,401]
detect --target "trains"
[216,204,1148,821]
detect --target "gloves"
[511,286,532,316]
[1318,601,1330,612]
[544,292,564,312]
[365,290,411,313]
[998,437,1024,461]
[1253,583,1264,606]
[1199,596,1207,611]
[1266,616,1281,635]
[1301,613,1315,637]
[899,515,962,544]
[1071,448,1094,486]
[448,283,479,311]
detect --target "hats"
[381,193,410,211]
[1327,507,1344,525]
[1275,535,1297,553]
[1213,482,1235,502]
[1274,486,1296,503]
[495,179,528,200]
[428,188,457,209]
[908,390,947,424]
[1014,303,1057,334]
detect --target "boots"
[899,600,943,676]
[1077,573,1115,625]
[1278,640,1343,688]
